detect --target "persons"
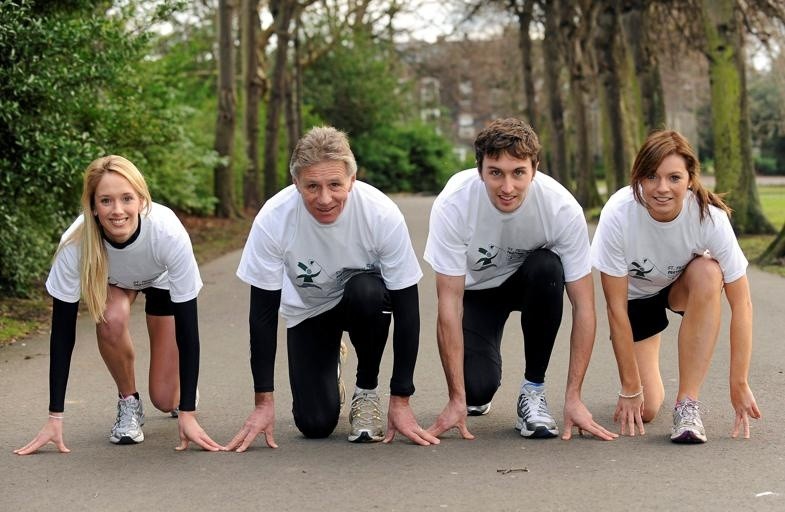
[225,124,441,450]
[589,130,761,445]
[425,118,618,444]
[10,153,227,455]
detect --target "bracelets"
[616,386,646,400]
[45,414,66,421]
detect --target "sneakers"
[107,393,146,446]
[346,384,387,445]
[467,404,492,417]
[668,398,710,445]
[513,379,560,441]
[170,381,200,419]
[338,340,347,410]
